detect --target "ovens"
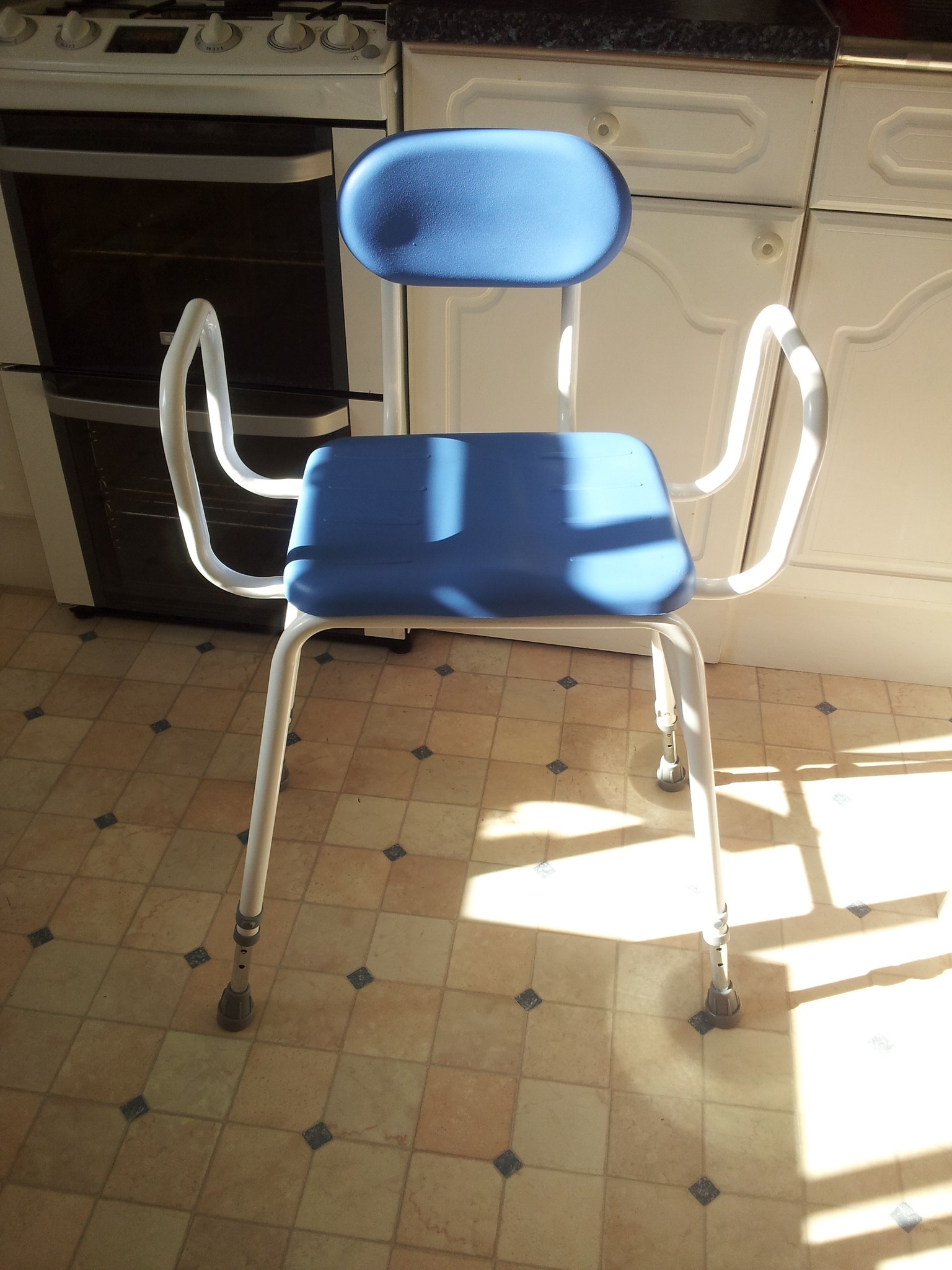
[0,11,418,658]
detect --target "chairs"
[154,122,832,1035]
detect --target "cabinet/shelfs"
[386,2,946,696]
